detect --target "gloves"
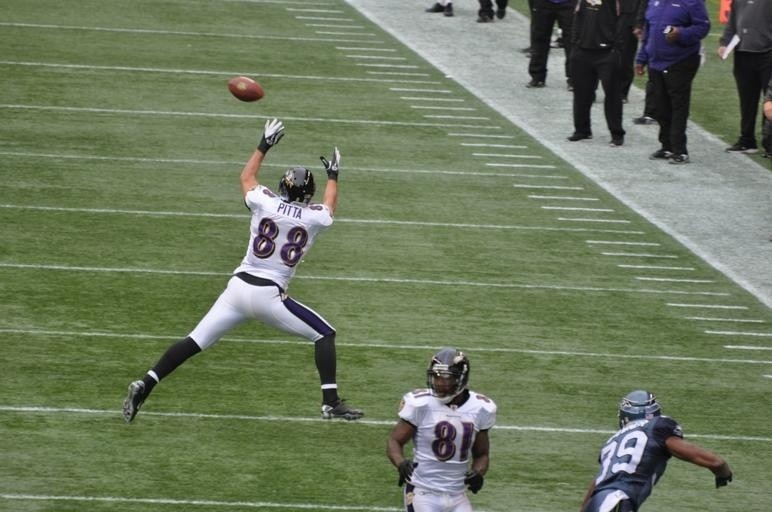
[715,471,732,488]
[257,118,285,156]
[397,460,419,486]
[320,147,341,181]
[464,469,483,493]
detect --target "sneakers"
[476,15,494,22]
[567,83,575,91]
[495,7,506,19]
[121,380,145,423]
[632,118,659,124]
[725,142,759,154]
[609,136,624,147]
[425,3,453,16]
[321,397,364,421]
[525,77,544,88]
[648,149,689,164]
[566,132,592,142]
[550,37,564,48]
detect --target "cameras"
[663,24,672,41]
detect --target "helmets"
[277,168,316,204]
[618,390,662,430]
[426,348,469,404]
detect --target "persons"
[580,390,732,512]
[386,348,498,512]
[528,0,771,165]
[427,1,509,22]
[123,117,365,422]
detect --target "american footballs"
[228,76,264,101]
